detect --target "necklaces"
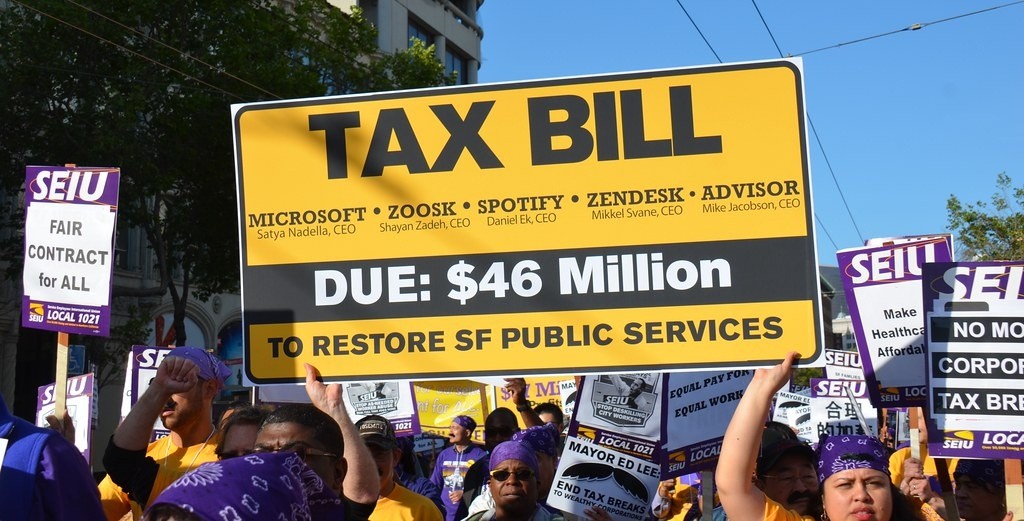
[164,424,215,474]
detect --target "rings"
[914,485,915,489]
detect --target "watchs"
[517,401,531,411]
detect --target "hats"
[953,459,1005,494]
[354,415,397,452]
[757,420,809,471]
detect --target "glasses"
[216,452,234,461]
[760,473,816,486]
[248,448,338,462]
[488,470,535,481]
[484,427,516,437]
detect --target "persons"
[608,374,646,412]
[0,344,1015,520]
